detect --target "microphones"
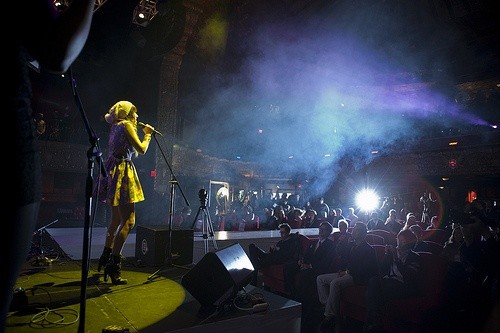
[138,122,163,137]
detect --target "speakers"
[181,242,255,307]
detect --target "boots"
[98,247,127,285]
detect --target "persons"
[165,189,500,333]
[93,101,155,285]
[0,0,97,333]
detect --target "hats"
[104,100,133,124]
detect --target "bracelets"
[142,134,151,142]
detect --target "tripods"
[148,132,192,281]
[191,200,218,254]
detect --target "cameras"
[199,189,208,199]
